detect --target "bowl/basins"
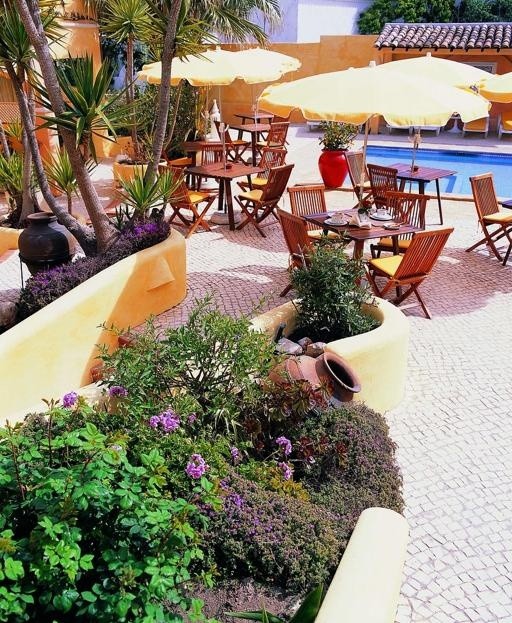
[226,164,233,169]
[410,166,419,171]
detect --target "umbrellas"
[137,49,282,169]
[376,52,495,171]
[236,46,302,115]
[478,71,512,104]
[213,46,297,140]
[258,61,490,208]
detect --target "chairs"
[497,111,512,141]
[461,115,490,138]
[275,148,457,321]
[468,173,512,266]
[158,106,290,240]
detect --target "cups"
[377,209,386,216]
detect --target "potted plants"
[318,118,360,187]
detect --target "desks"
[447,116,461,134]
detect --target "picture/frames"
[459,61,496,75]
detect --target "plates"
[372,213,389,219]
[324,219,347,226]
[383,223,402,229]
